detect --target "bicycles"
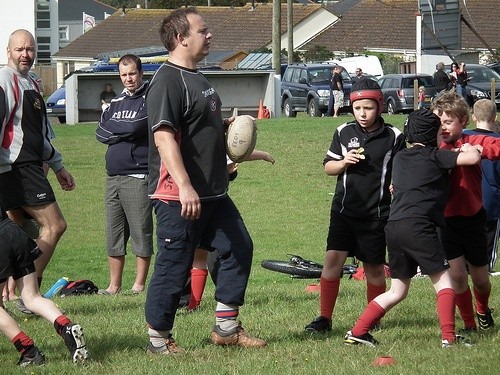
[264,250,361,280]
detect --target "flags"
[84,14,95,27]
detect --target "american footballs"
[225,116,257,162]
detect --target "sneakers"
[63,323,89,364]
[455,327,477,340]
[212,321,267,348]
[347,331,383,348]
[476,307,496,332]
[304,315,332,334]
[147,337,183,356]
[14,298,32,314]
[16,346,45,368]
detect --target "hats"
[349,78,383,118]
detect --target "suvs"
[281,61,356,117]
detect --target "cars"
[376,74,436,114]
[434,63,500,111]
[46,83,66,124]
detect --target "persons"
[1,276,20,301]
[100,83,118,112]
[342,111,481,348]
[331,66,344,117]
[430,91,495,338]
[326,67,338,117]
[95,55,155,294]
[304,79,402,335]
[430,61,468,100]
[180,149,276,309]
[460,98,500,273]
[418,86,427,109]
[0,207,89,367]
[0,30,75,315]
[143,9,266,358]
[352,68,367,86]
